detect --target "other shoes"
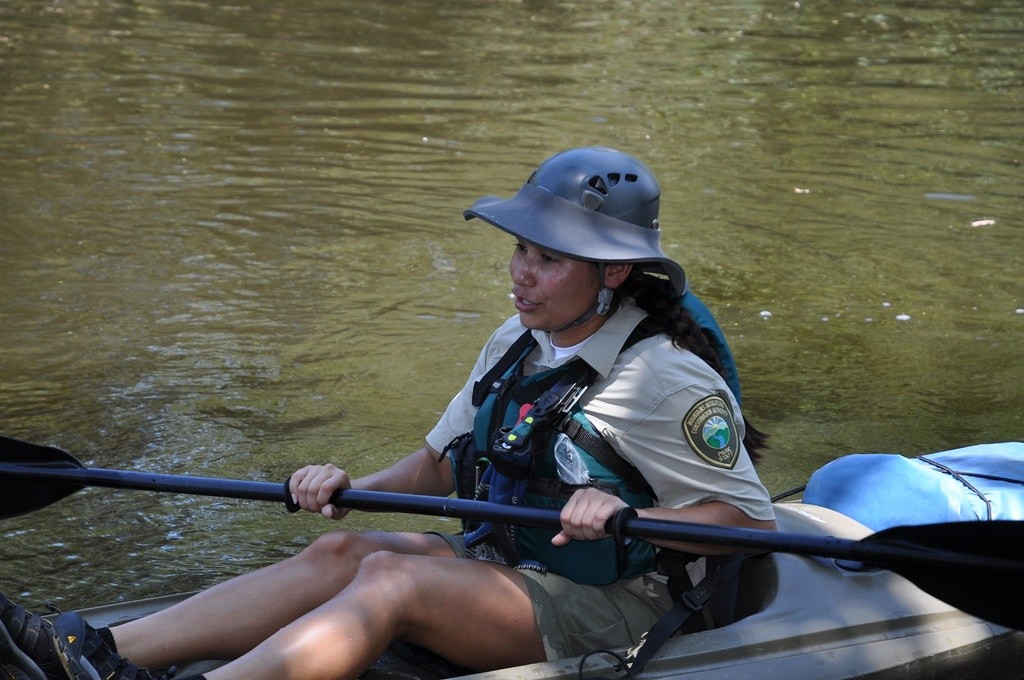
[0,591,204,680]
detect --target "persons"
[1,147,779,680]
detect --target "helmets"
[462,146,691,298]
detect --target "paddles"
[1,433,1024,633]
